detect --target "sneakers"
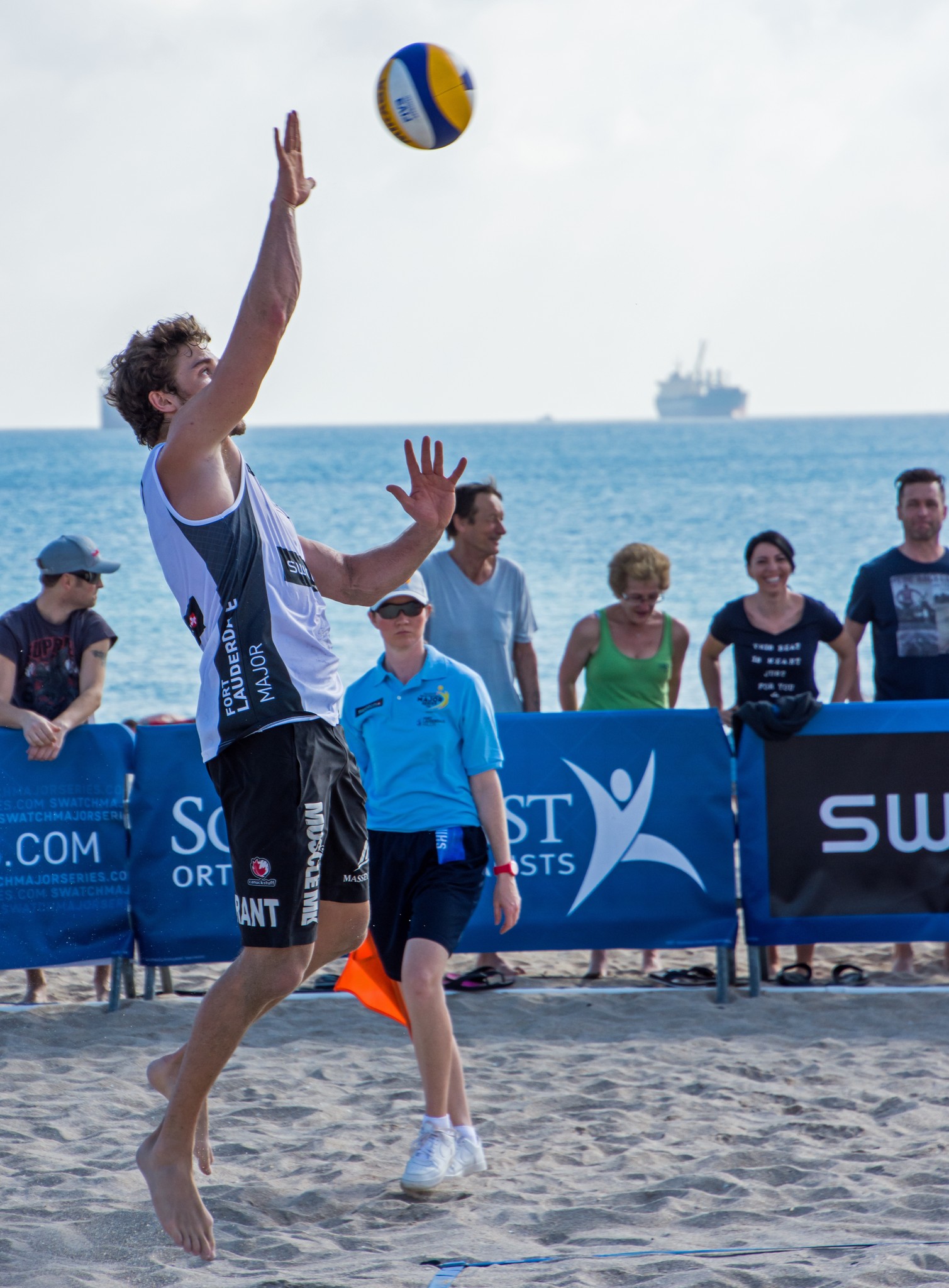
[400,1120,457,1190]
[444,1138,486,1179]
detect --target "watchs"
[493,860,519,876]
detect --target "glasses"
[376,601,424,620]
[71,572,97,584]
[623,591,663,606]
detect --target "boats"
[654,336,747,419]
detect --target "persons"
[845,469,949,978]
[343,571,520,1186]
[102,108,468,1258]
[558,542,690,979]
[416,481,540,975]
[1,535,120,1003]
[698,530,858,980]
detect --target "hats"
[38,535,120,573]
[368,570,430,611]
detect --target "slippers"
[832,963,866,987]
[777,963,812,987]
[648,967,716,986]
[443,966,515,991]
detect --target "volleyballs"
[376,42,476,151]
